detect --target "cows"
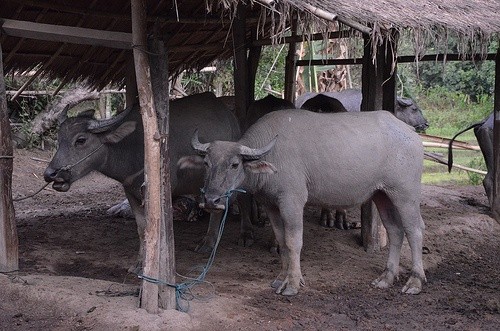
[234,88,429,249]
[44,91,242,280]
[447,109,494,208]
[191,108,427,296]
